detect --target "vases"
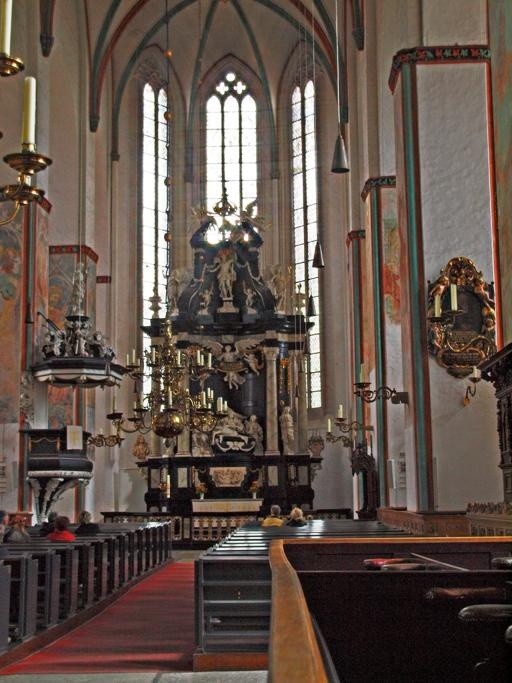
[200,493,204,500]
[252,492,256,500]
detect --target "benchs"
[192,518,423,652]
[0,521,173,653]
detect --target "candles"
[21,75,36,146]
[328,419,331,432]
[434,284,458,317]
[338,404,343,419]
[0,0,14,58]
[360,363,367,382]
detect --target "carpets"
[0,561,195,673]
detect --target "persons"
[45,515,77,542]
[243,287,257,307]
[74,509,100,537]
[428,275,449,317]
[2,514,32,543]
[246,415,264,453]
[243,352,260,376]
[285,506,307,527]
[39,510,59,535]
[202,254,237,296]
[260,504,285,528]
[0,509,12,543]
[200,288,214,305]
[279,407,295,453]
[215,344,240,390]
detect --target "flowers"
[249,481,260,492]
[195,482,208,494]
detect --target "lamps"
[302,1,325,318]
[329,1,350,174]
[99,319,233,458]
